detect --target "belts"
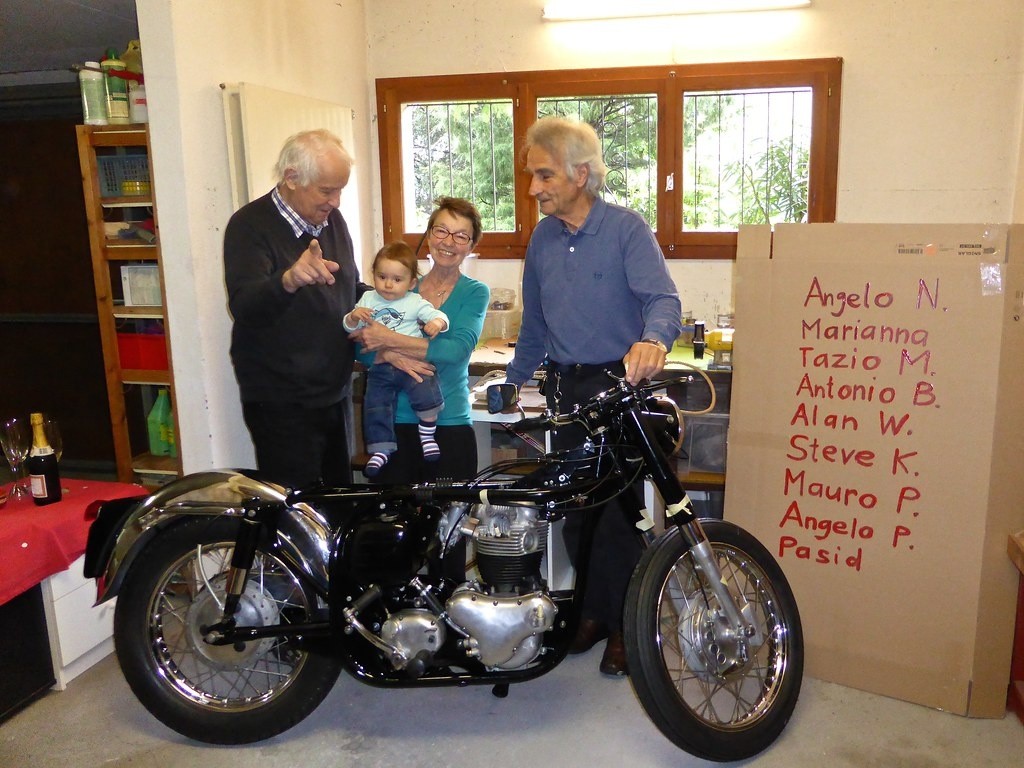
[547,361,619,374]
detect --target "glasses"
[432,226,473,245]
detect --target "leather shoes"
[599,631,628,676]
[565,615,604,655]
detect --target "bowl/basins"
[487,288,517,311]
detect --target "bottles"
[147,390,176,458]
[78,49,148,125]
[28,413,62,505]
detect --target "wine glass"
[0,418,30,499]
[44,421,69,494]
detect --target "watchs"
[642,339,667,353]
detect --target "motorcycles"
[81,376,805,764]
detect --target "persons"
[225,129,375,659]
[356,199,489,583]
[500,118,682,675]
[343,244,449,479]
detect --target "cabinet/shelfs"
[351,335,733,590]
[76,124,183,495]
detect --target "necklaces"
[438,291,446,297]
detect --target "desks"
[0,474,151,727]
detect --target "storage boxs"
[720,224,1023,718]
[97,155,151,197]
[480,307,521,339]
[668,382,730,474]
